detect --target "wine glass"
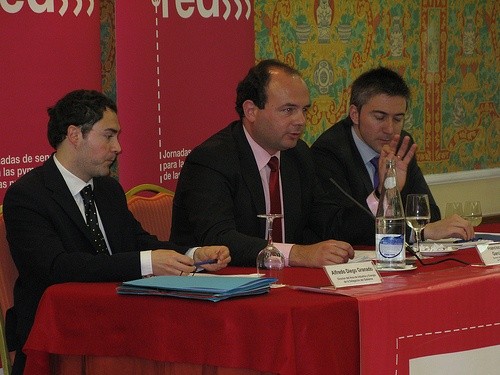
[405,194,433,260]
[256,215,285,282]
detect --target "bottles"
[375,160,405,271]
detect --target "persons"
[3,89,231,375]
[309,68,475,246]
[169,58,417,268]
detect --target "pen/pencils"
[193,257,219,267]
[452,237,484,243]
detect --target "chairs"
[125,183,175,241]
[0,205,20,375]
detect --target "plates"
[419,247,459,256]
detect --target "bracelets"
[375,187,380,199]
[421,229,425,241]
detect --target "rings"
[398,155,400,159]
[180,271,183,276]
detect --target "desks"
[21,222,500,375]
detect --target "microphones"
[317,167,418,255]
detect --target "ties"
[370,155,381,191]
[266,156,283,244]
[81,185,111,256]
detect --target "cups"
[462,200,482,226]
[445,202,463,218]
